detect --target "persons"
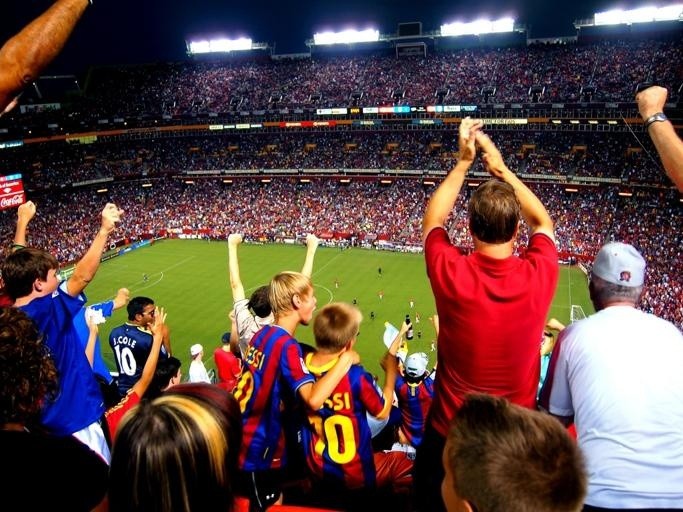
[410,116,561,512]
[0,0,92,114]
[1,40,682,334]
[0,199,594,512]
[538,242,683,512]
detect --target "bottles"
[406,314,414,340]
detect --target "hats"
[222,333,230,343]
[592,242,646,287]
[406,352,428,377]
[190,344,203,355]
